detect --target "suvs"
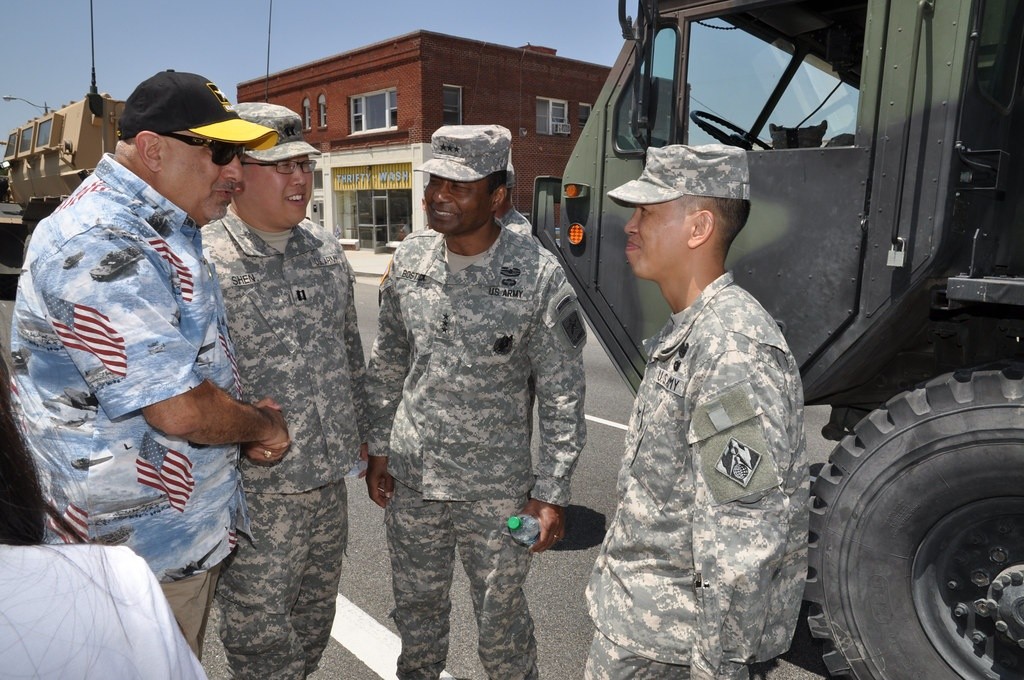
[530,0,1024,680]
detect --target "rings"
[554,535,559,539]
[264,450,272,459]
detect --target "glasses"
[156,131,246,167]
[241,160,317,175]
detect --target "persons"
[585,143,810,680]
[0,67,586,680]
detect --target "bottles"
[508,514,541,545]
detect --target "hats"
[606,143,750,209]
[232,102,322,162]
[118,70,279,152]
[414,124,512,183]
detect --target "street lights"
[2,96,51,117]
